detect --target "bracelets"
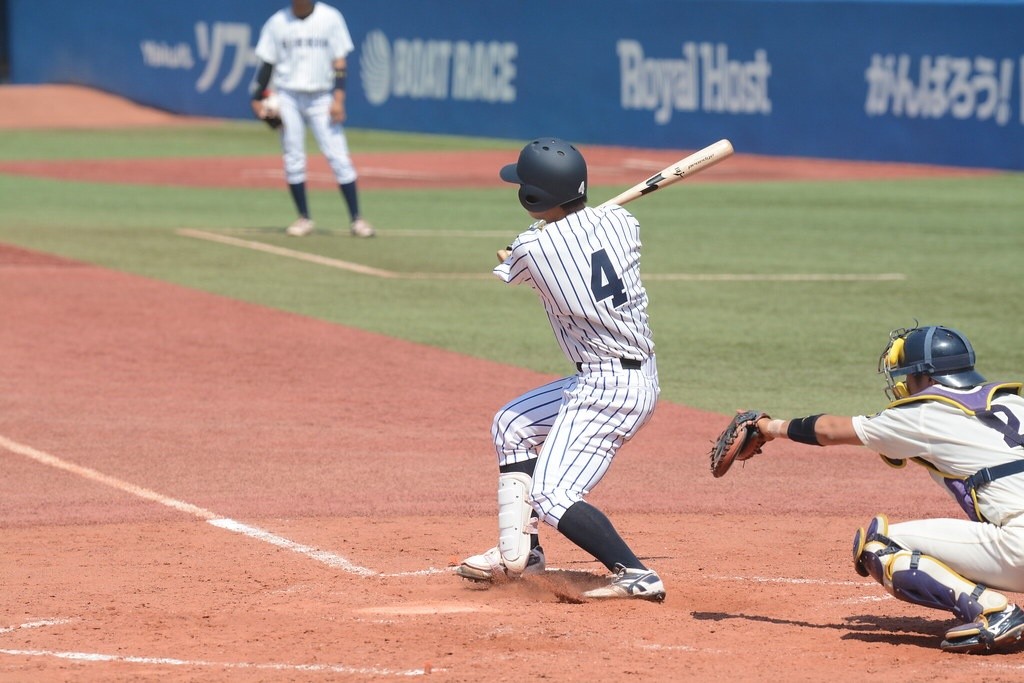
[767,420,785,438]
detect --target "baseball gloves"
[707,408,771,478]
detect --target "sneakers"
[582,563,666,602]
[457,545,545,579]
[940,604,1024,653]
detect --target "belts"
[576,358,641,372]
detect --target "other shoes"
[286,216,313,236]
[351,214,374,237]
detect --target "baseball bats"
[496,135,736,267]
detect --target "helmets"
[500,137,587,212]
[904,327,987,388]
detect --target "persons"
[457,138,667,602]
[710,325,1024,651]
[248,0,374,238]
